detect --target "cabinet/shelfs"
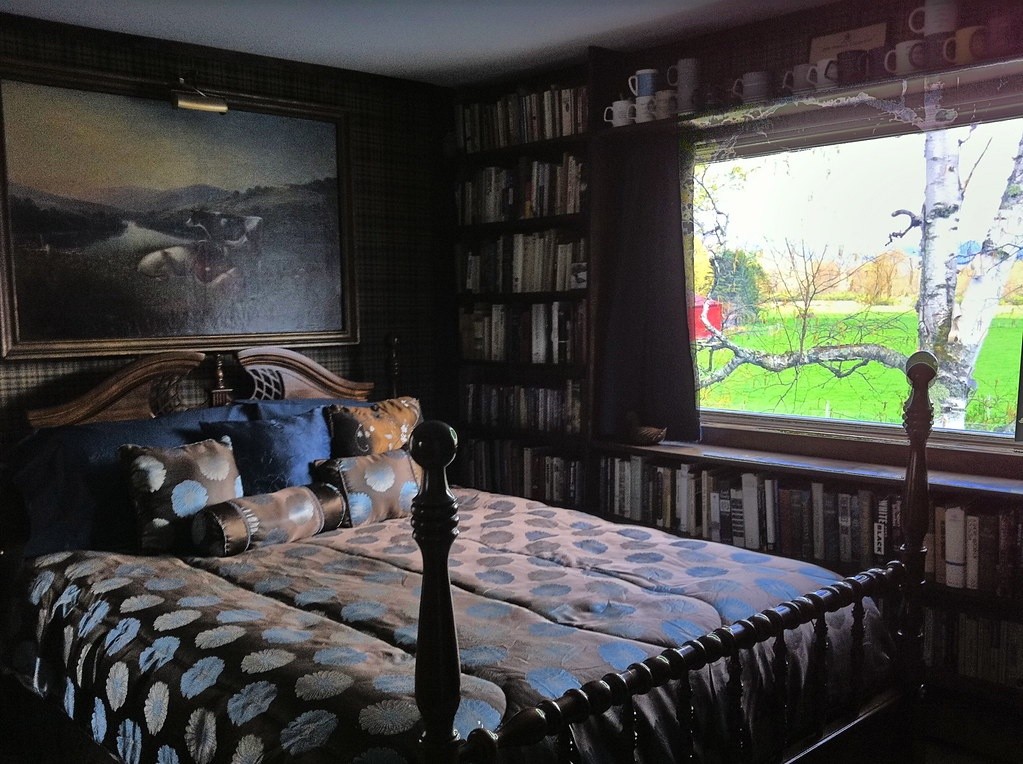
[427,85,1023,703]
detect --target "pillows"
[324,394,427,454]
[115,435,254,562]
[197,407,335,497]
[317,450,423,530]
[190,478,346,562]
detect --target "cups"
[968,15,1023,63]
[627,97,657,123]
[910,0,961,36]
[859,46,895,80]
[668,58,702,88]
[910,29,956,72]
[733,71,772,104]
[647,90,677,120]
[782,64,818,95]
[603,101,635,127]
[806,58,839,91]
[942,24,989,68]
[628,69,659,95]
[669,88,703,119]
[824,49,868,86]
[885,38,925,76]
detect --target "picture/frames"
[0,54,367,369]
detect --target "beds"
[1,346,939,762]
